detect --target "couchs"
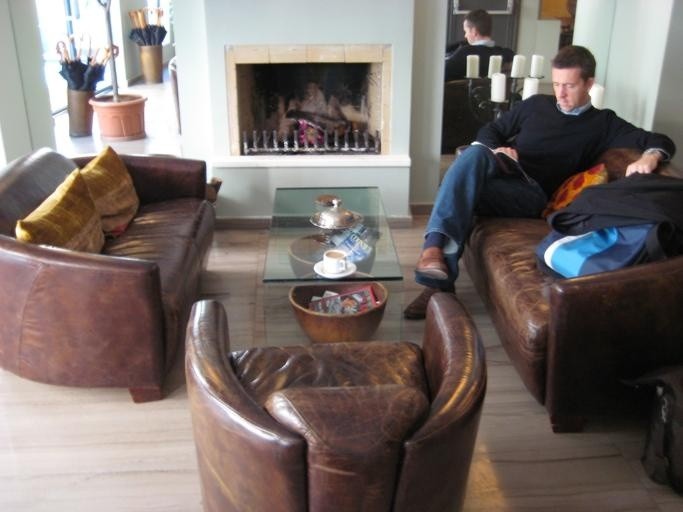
[0,145,222,405]
[185,290,489,512]
[439,143,683,434]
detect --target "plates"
[308,210,362,230]
[313,260,356,279]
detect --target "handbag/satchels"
[613,364,682,489]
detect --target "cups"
[322,249,348,274]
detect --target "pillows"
[15,165,105,253]
[78,145,140,241]
[545,163,607,215]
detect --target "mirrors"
[440,0,580,191]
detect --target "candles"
[523,78,539,99]
[531,55,545,80]
[466,55,479,78]
[512,54,526,79]
[489,54,503,79]
[588,84,604,109]
[491,73,506,104]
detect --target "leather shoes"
[414,247,449,281]
[403,286,442,320]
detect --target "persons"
[403,48,677,318]
[445,9,517,81]
